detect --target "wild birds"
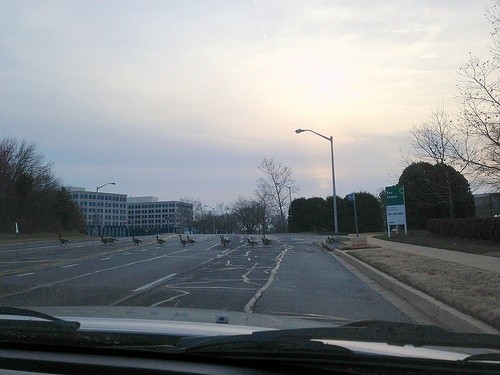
[100,236,114,245]
[247,233,272,247]
[219,235,231,246]
[156,236,166,247]
[131,234,141,246]
[57,233,70,247]
[179,234,195,247]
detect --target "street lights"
[275,183,292,221]
[95,182,115,235]
[295,129,338,236]
